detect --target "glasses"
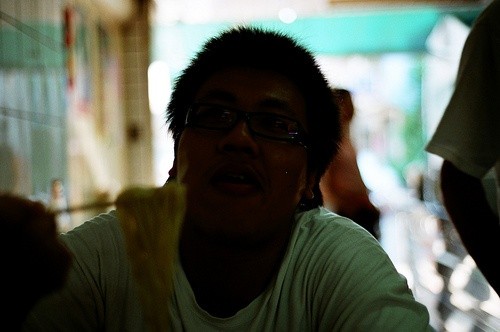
[180,104,311,154]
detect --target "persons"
[0,27,434,332]
[424,0,500,296]
